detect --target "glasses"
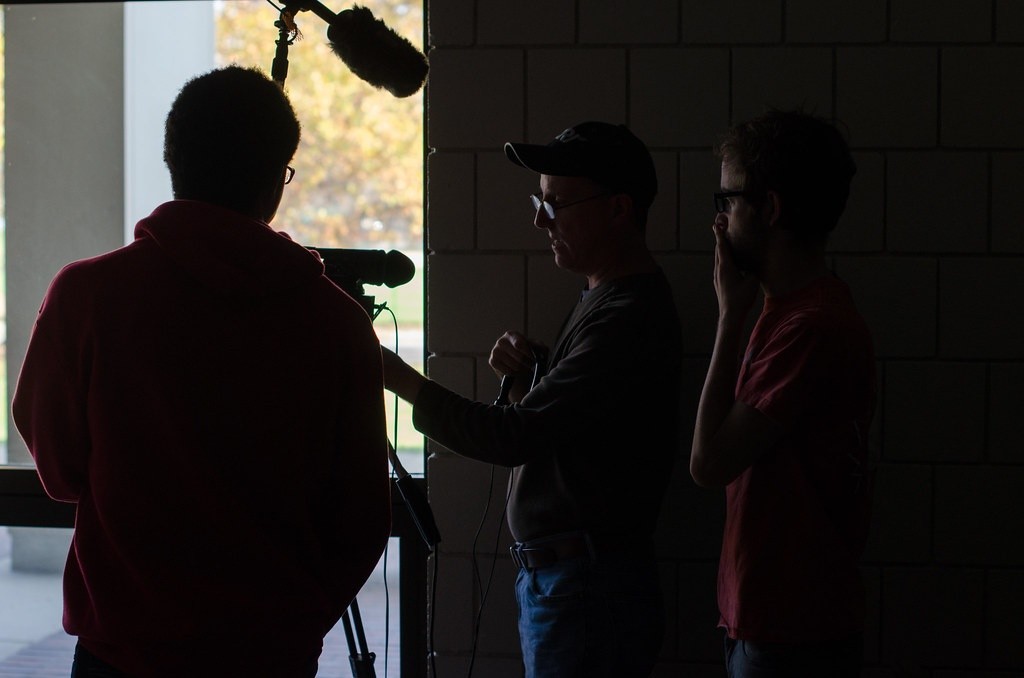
[529,192,608,219]
[714,191,745,213]
[283,165,294,184]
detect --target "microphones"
[280,0,430,97]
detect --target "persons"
[690,111,876,678]
[11,67,392,678]
[380,120,682,678]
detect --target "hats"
[504,121,658,205]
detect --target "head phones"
[493,342,546,405]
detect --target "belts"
[509,530,627,570]
